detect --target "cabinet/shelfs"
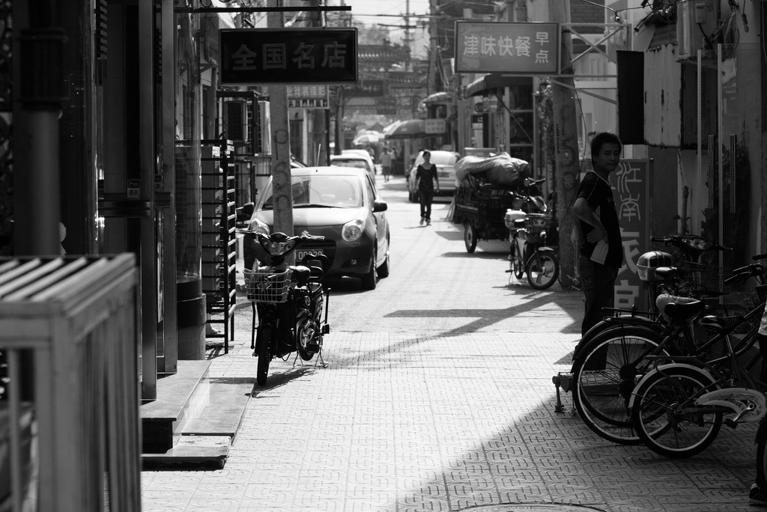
[173,135,239,356]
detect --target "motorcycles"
[235,227,332,387]
[502,209,562,291]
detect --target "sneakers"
[420,217,424,225]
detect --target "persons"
[413,150,439,226]
[568,129,623,372]
[366,142,399,182]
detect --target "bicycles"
[626,313,766,460]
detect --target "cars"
[327,153,376,186]
[406,149,463,204]
[240,164,391,292]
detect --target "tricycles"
[451,174,554,256]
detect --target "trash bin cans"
[175,275,206,359]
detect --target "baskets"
[243,268,291,303]
[526,213,552,241]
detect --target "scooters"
[635,233,735,366]
